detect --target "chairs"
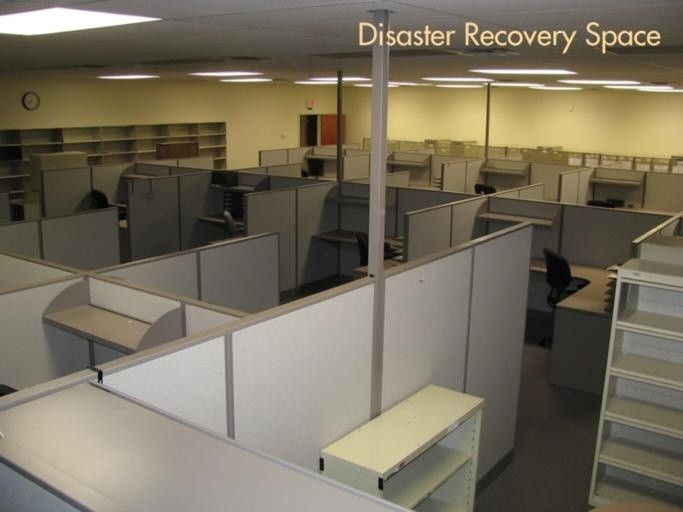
[587,200,613,208]
[223,210,244,236]
[474,184,496,194]
[355,231,390,266]
[91,189,127,228]
[538,248,579,347]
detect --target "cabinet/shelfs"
[137,122,227,169]
[587,257,683,511]
[320,381,486,510]
[63,125,137,166]
[0,120,63,200]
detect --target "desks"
[530,257,616,395]
[112,201,128,207]
[198,212,243,225]
[313,229,403,279]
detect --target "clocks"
[21,92,39,111]
